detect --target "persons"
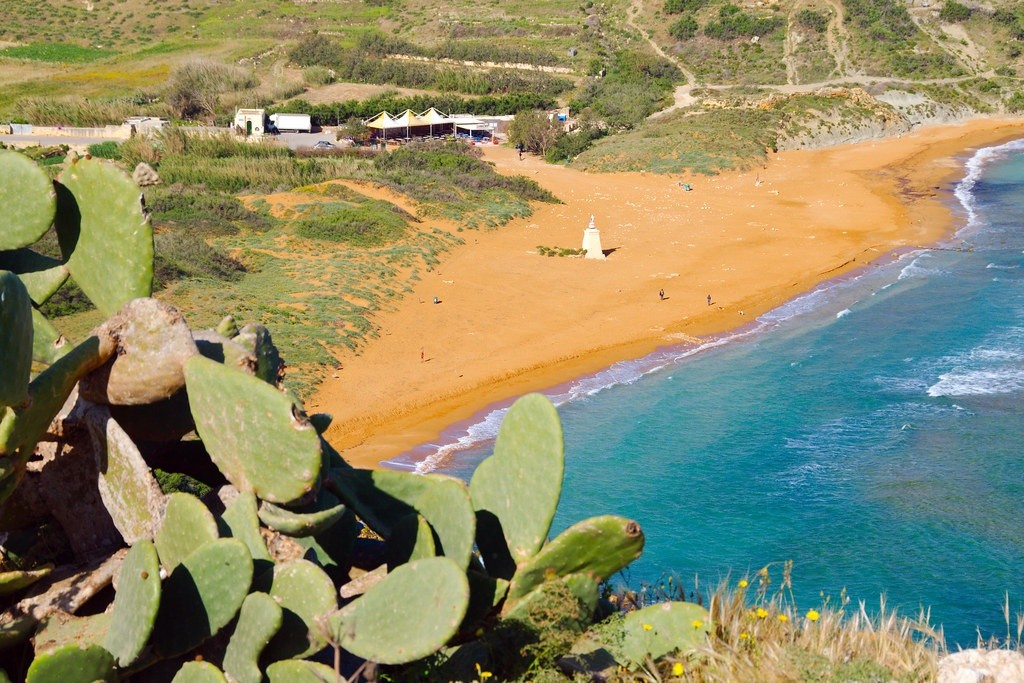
[707,294,712,306]
[660,288,664,300]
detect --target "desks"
[372,130,490,145]
